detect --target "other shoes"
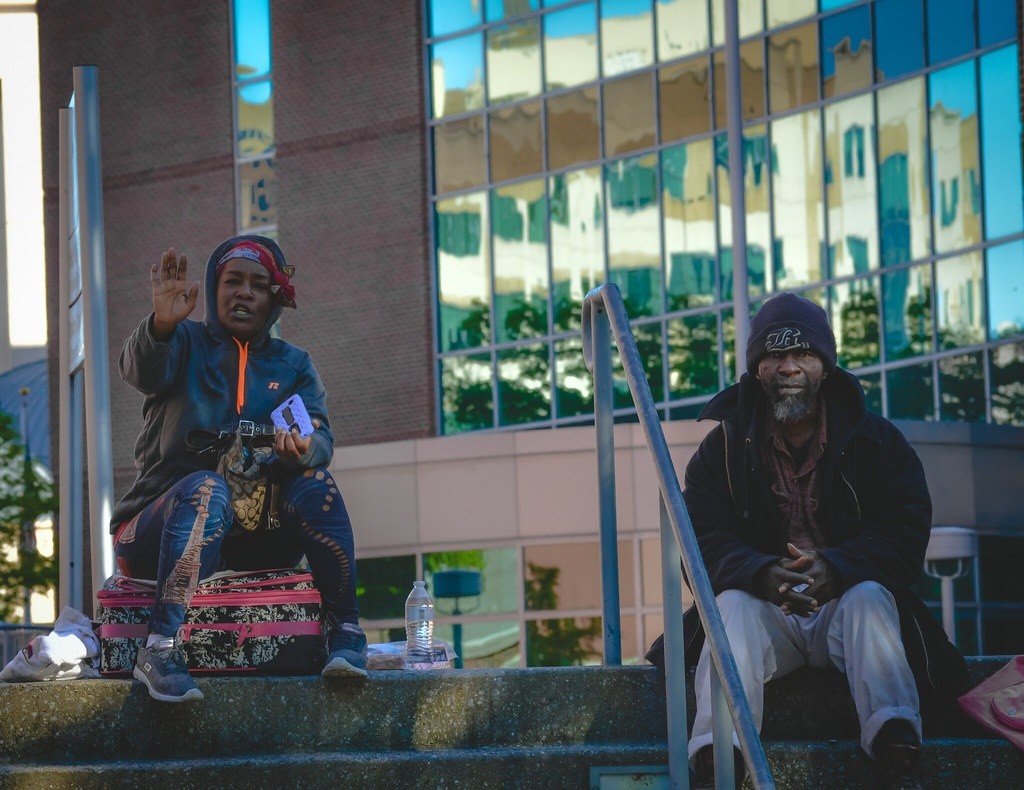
[692,744,744,790]
[871,740,925,790]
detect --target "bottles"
[405,581,435,670]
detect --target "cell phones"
[270,394,315,440]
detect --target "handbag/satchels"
[956,654,1024,752]
[367,639,460,670]
[0,605,103,680]
[182,420,292,536]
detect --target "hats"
[745,292,837,378]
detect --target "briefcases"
[95,563,330,678]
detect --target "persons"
[108,234,369,703]
[642,292,966,790]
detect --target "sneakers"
[321,610,368,678]
[132,635,205,703]
